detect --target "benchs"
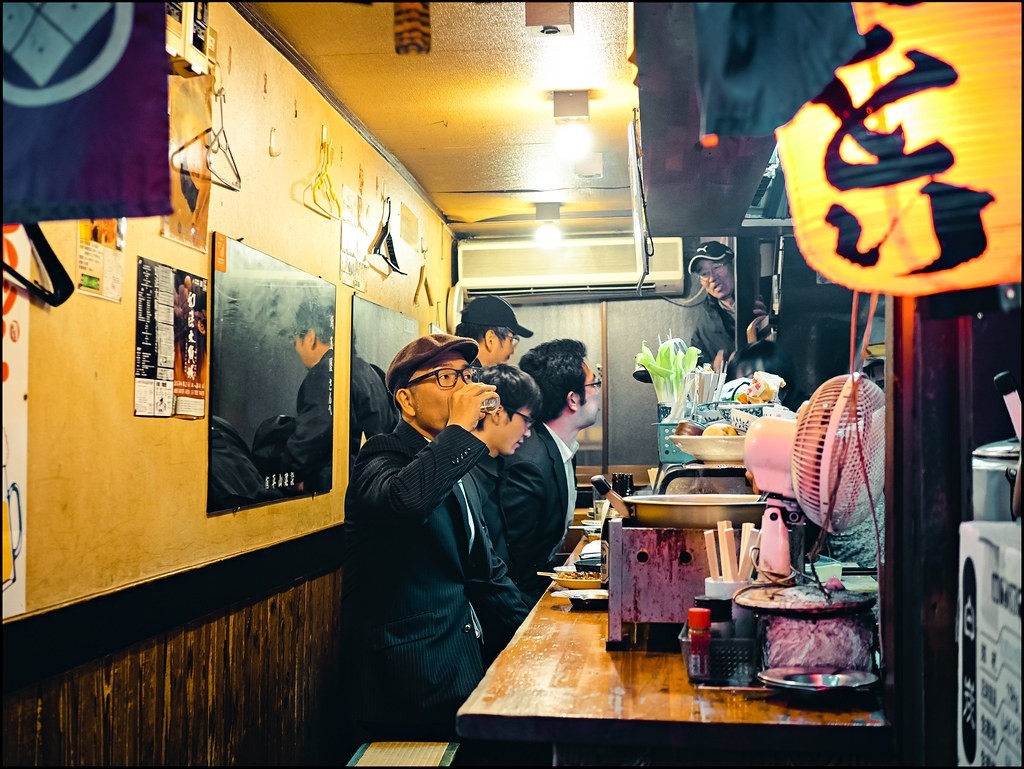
[345,742,460,767]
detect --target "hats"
[384,334,479,395]
[278,296,339,340]
[461,296,533,338]
[688,241,735,275]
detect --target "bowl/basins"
[575,560,602,574]
[731,410,759,435]
[719,404,774,423]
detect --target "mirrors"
[349,294,420,482]
[504,293,609,476]
[205,231,336,515]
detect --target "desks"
[455,508,889,769]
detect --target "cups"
[480,395,500,413]
[705,576,752,598]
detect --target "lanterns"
[774,1,1022,298]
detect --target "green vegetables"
[637,343,701,406]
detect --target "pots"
[592,474,770,528]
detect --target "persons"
[453,294,532,368]
[208,293,398,515]
[687,241,767,375]
[331,335,530,742]
[466,364,543,600]
[498,337,602,607]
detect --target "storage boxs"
[958,519,1021,767]
[678,619,759,684]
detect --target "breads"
[737,372,776,403]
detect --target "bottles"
[694,596,735,640]
[686,609,712,679]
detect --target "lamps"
[553,90,595,160]
[518,168,573,255]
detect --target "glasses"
[503,404,536,428]
[585,378,601,389]
[405,367,481,387]
[698,260,730,281]
[289,329,310,346]
[495,331,520,347]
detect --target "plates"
[669,436,745,460]
[552,576,601,589]
[551,590,609,609]
[759,667,878,693]
[554,566,576,574]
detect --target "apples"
[701,424,739,437]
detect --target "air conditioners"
[457,237,686,305]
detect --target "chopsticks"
[704,518,760,582]
[688,373,727,403]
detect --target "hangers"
[312,138,341,222]
[414,246,437,309]
[205,86,243,193]
[0,220,74,308]
[368,198,408,278]
